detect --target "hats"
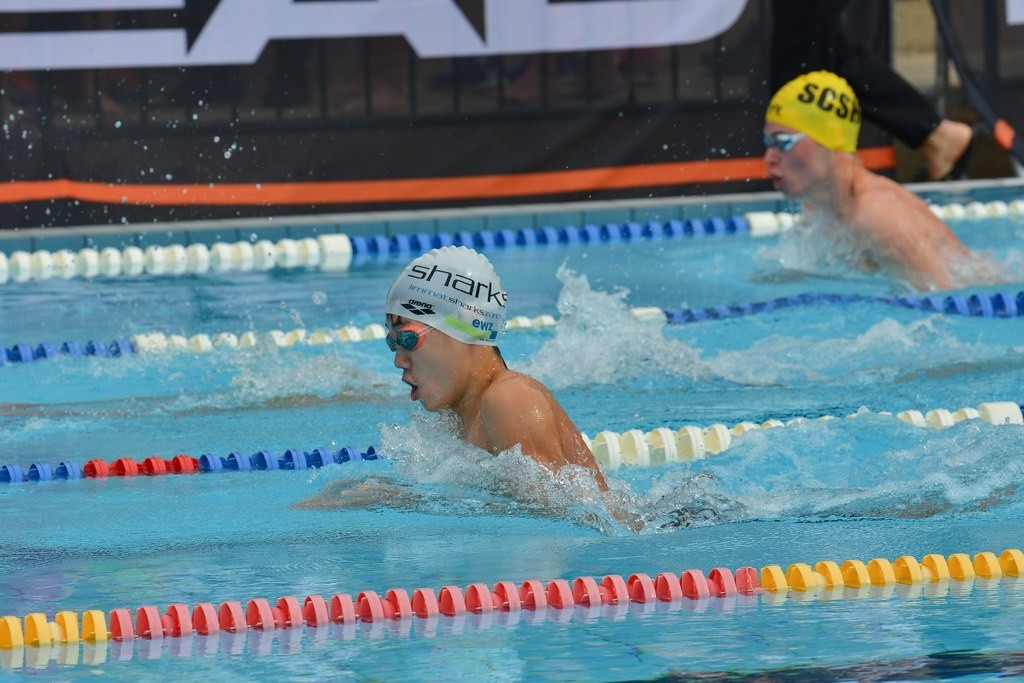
[385,245,507,346]
[766,70,860,153]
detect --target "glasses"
[385,326,434,352]
[762,133,803,153]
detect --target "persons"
[382,244,612,495]
[762,69,970,264]
[770,0,973,182]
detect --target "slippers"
[932,122,986,180]
